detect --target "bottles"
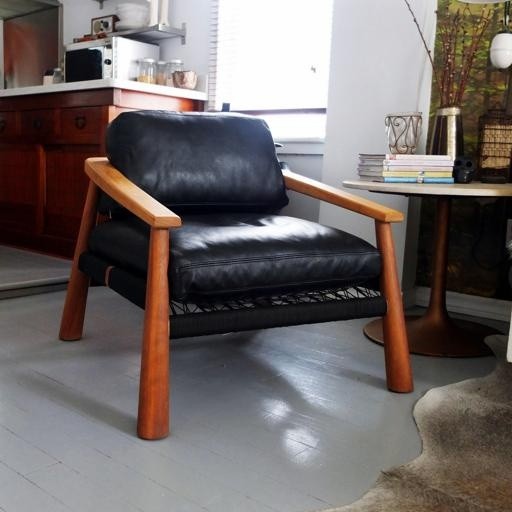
[136,59,184,87]
[52,68,64,85]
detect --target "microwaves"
[63,36,159,83]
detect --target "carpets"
[314,325,511,511]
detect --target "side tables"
[339,169,512,360]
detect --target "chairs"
[57,108,418,439]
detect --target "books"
[356,151,455,184]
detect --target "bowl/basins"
[172,70,197,89]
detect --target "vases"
[427,104,466,169]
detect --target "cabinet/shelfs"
[1,92,203,261]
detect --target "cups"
[43,76,53,85]
